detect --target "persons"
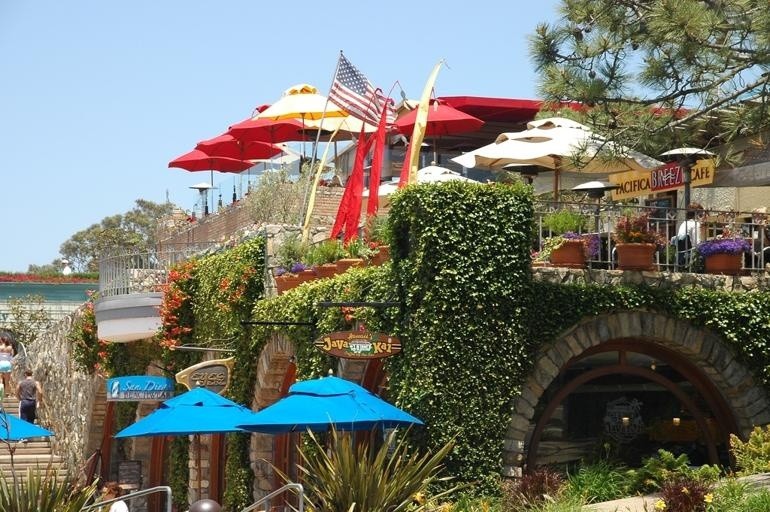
[677,202,703,273]
[62,259,73,277]
[14,369,43,444]
[101,481,130,512]
[0,336,14,399]
[189,498,223,512]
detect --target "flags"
[328,52,396,129]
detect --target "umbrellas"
[166,81,349,214]
[358,162,483,198]
[449,112,669,244]
[111,381,260,502]
[390,101,484,166]
[235,368,426,512]
[0,406,55,441]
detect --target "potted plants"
[270,207,391,298]
[545,207,591,269]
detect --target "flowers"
[612,211,668,245]
[694,237,751,256]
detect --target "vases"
[705,253,744,275]
[615,238,656,268]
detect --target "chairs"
[671,233,699,272]
